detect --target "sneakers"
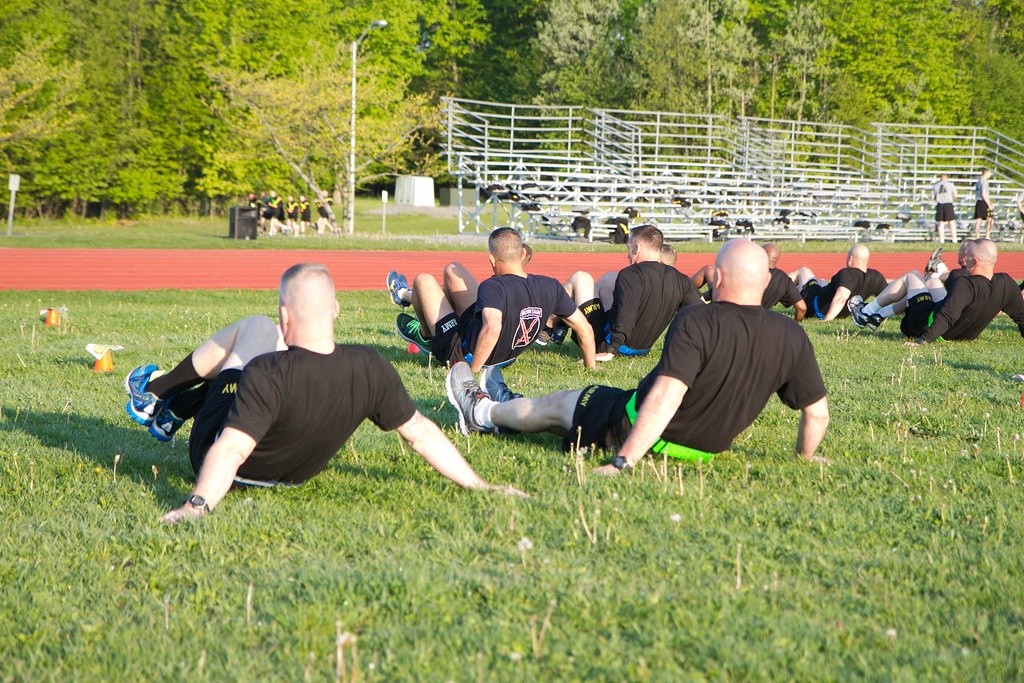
[386,270,413,307]
[123,363,186,442]
[396,313,434,356]
[445,361,523,437]
[923,247,944,281]
[847,295,885,331]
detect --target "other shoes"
[535,324,568,346]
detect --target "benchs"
[456,152,1024,245]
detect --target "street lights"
[342,20,392,240]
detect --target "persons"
[975,167,994,240]
[385,243,531,310]
[787,244,888,322]
[124,263,527,525]
[661,243,677,267]
[445,237,830,478]
[248,189,333,237]
[1018,191,1024,222]
[924,239,967,288]
[395,226,596,372]
[534,225,705,363]
[846,238,1024,345]
[934,174,957,242]
[690,243,807,322]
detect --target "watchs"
[609,455,632,472]
[185,495,209,515]
